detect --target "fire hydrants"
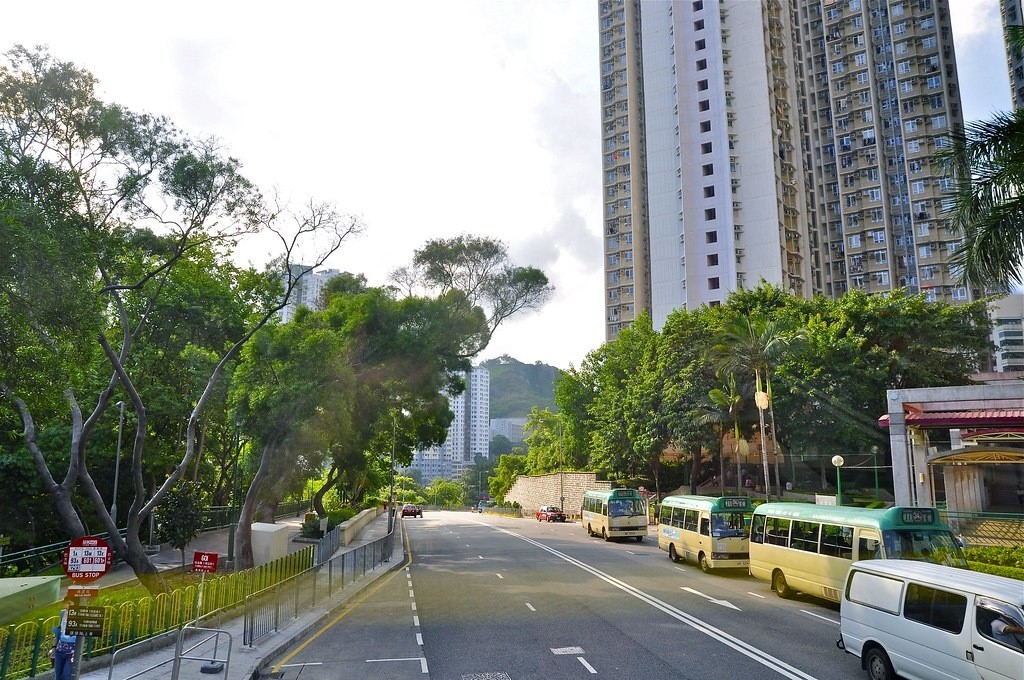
[471,505,474,513]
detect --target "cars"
[535,506,566,522]
[401,504,417,518]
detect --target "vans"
[836,560,1024,680]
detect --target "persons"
[551,508,554,511]
[755,526,849,556]
[991,615,1024,651]
[921,548,929,558]
[384,502,387,512]
[729,520,738,528]
[650,501,660,524]
[713,467,793,492]
[875,532,899,558]
[471,506,474,512]
[418,503,423,518]
[393,506,396,517]
[712,517,726,529]
[48,596,80,680]
[612,506,617,516]
[535,510,539,520]
[623,503,636,515]
[479,500,482,513]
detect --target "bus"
[658,495,756,573]
[749,506,969,604]
[581,488,647,542]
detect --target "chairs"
[768,530,851,557]
[663,515,698,531]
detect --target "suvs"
[478,493,496,513]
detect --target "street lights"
[387,394,403,535]
[832,456,844,506]
[539,418,563,510]
[701,406,724,496]
[479,471,489,497]
[871,446,879,501]
[108,401,125,549]
